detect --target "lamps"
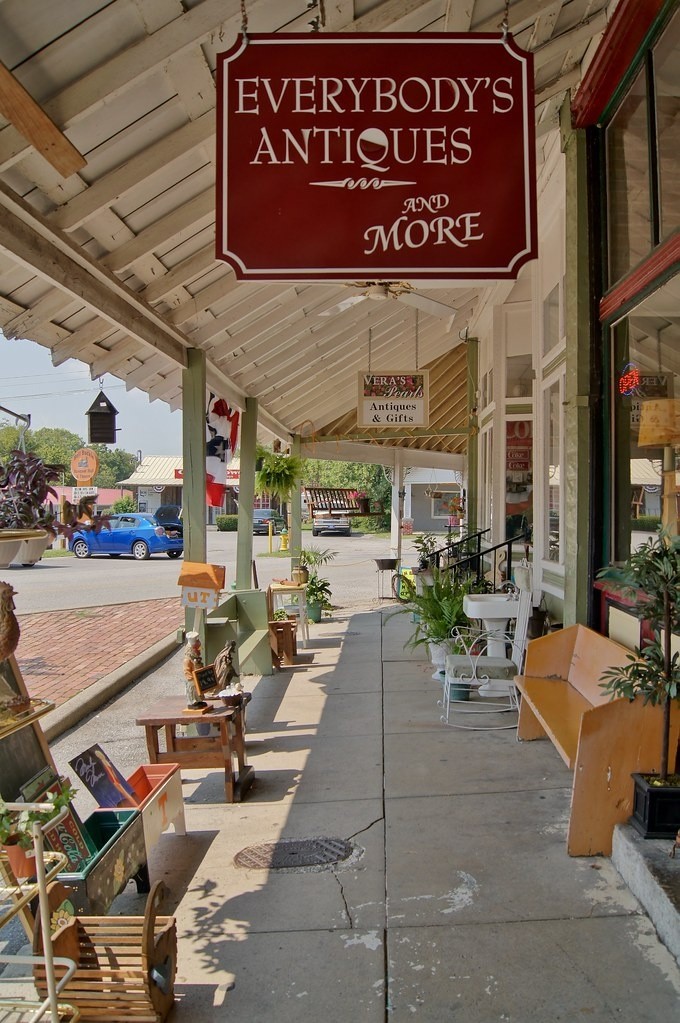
[638,399,680,471]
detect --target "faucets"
[497,580,520,601]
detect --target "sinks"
[462,594,519,618]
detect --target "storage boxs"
[51,763,186,935]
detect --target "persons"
[184,631,208,710]
[211,640,239,695]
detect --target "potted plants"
[255,440,308,505]
[381,564,491,666]
[596,521,679,839]
[0,776,79,877]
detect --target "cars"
[69,505,184,560]
[312,502,351,536]
[252,509,288,535]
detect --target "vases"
[307,601,322,623]
[447,515,461,526]
[356,499,370,513]
[439,671,471,701]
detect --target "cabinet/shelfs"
[0,797,80,1023]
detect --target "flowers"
[346,491,369,501]
[293,575,333,624]
[445,636,481,656]
[449,496,462,516]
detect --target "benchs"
[205,591,273,694]
[514,623,680,857]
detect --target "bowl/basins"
[374,558,401,570]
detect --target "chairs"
[265,586,298,665]
[437,591,533,731]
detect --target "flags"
[205,389,240,508]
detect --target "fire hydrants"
[279,529,289,551]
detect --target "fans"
[264,279,497,319]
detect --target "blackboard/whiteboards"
[193,663,219,695]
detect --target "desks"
[269,582,310,649]
[135,692,255,803]
